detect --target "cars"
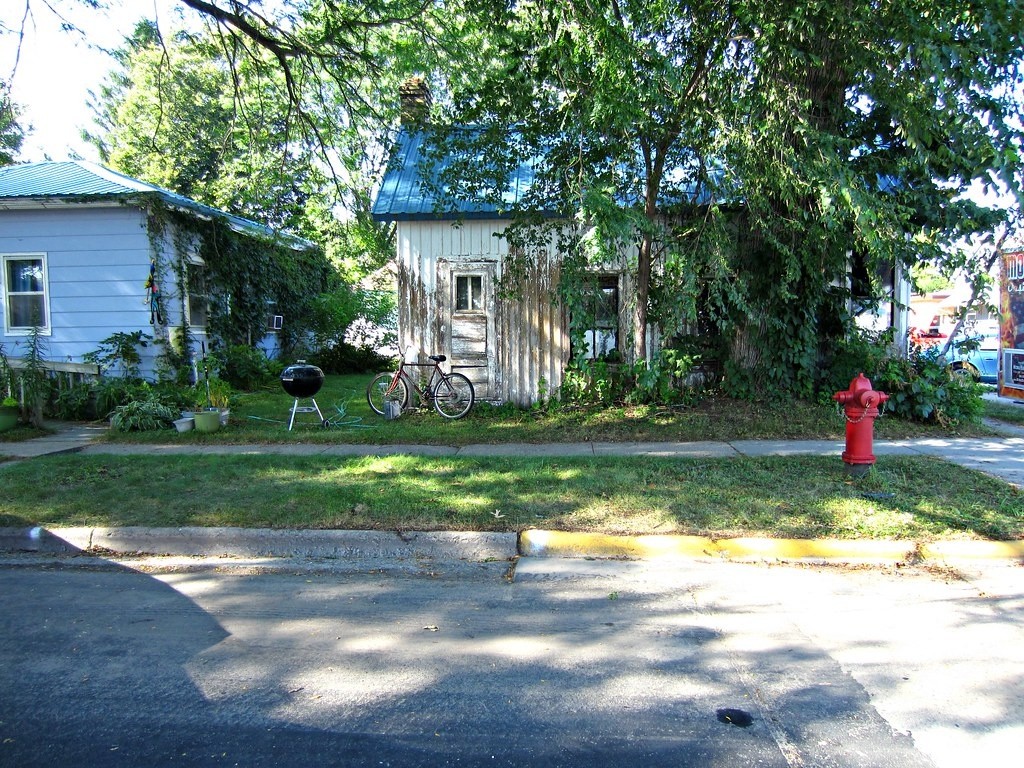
[928,331,999,386]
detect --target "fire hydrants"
[832,371,893,465]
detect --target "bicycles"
[365,341,475,420]
